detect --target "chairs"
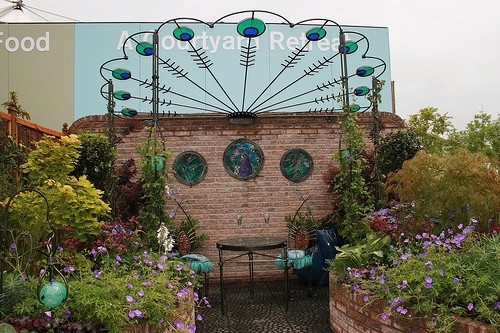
[170,199,215,295]
[276,195,318,300]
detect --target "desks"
[216,237,288,314]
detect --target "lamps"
[0,188,68,307]
